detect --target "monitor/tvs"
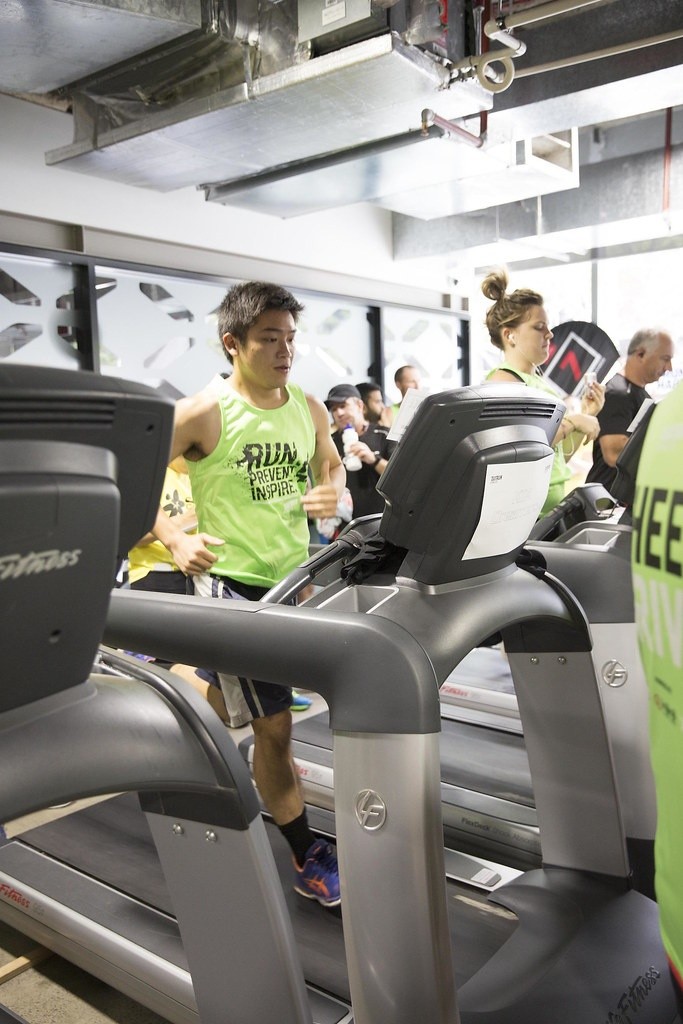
[0,363,658,712]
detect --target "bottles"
[342,423,363,472]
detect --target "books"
[386,387,432,442]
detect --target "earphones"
[508,334,516,345]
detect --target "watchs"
[369,454,383,468]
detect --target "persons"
[323,384,410,586]
[355,382,394,428]
[390,366,423,418]
[483,265,605,524]
[584,327,675,494]
[150,282,347,907]
[129,455,316,729]
[631,375,683,1024]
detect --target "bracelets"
[560,417,577,440]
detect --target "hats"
[323,383,361,409]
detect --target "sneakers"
[289,690,311,710]
[290,838,341,908]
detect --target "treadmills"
[0,360,681,1024]
